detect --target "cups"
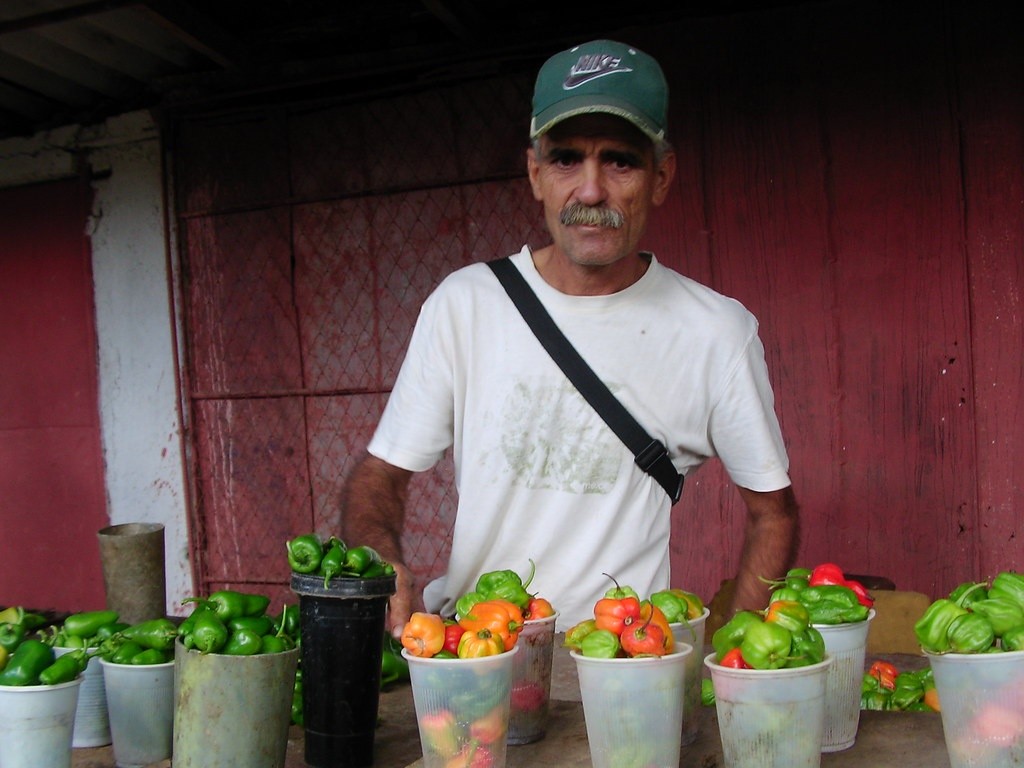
[644,606,713,746]
[0,616,306,768]
[400,643,522,768]
[570,641,694,768]
[499,610,561,745]
[800,607,878,753]
[920,643,1024,768]
[701,649,832,767]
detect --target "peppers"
[860,659,939,712]
[284,534,395,589]
[402,560,556,768]
[562,573,717,768]
[709,599,828,768]
[912,572,1024,768]
[756,562,876,625]
[0,590,409,730]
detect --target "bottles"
[289,573,398,768]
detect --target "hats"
[530,39,667,148]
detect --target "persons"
[340,40,802,703]
